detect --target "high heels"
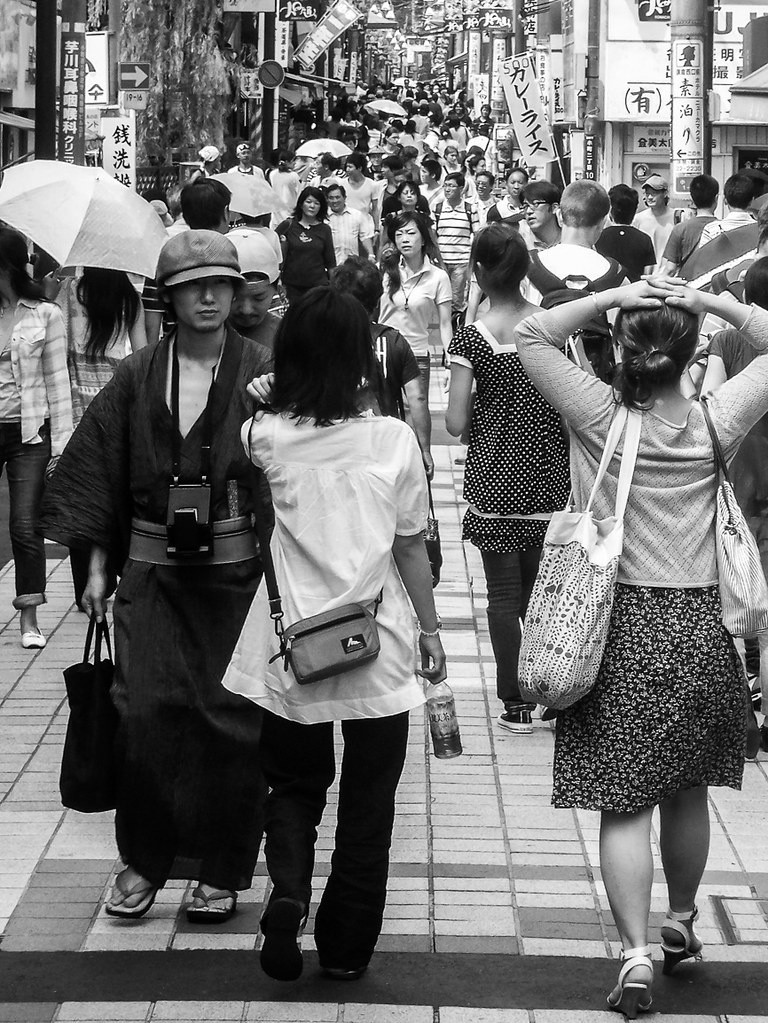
[660,904,702,975]
[606,944,653,1018]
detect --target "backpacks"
[525,249,627,385]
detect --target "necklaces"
[400,271,425,310]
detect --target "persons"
[0,80,768,1021]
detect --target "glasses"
[519,200,550,211]
[442,184,459,189]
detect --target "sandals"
[186,888,238,920]
[106,870,158,919]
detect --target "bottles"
[425,682,462,759]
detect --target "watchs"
[368,255,376,258]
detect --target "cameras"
[162,503,215,566]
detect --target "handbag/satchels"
[59,608,121,814]
[517,402,643,710]
[700,400,768,639]
[268,603,380,685]
[423,474,442,589]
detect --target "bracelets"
[417,614,443,636]
[592,293,602,316]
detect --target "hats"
[155,229,245,287]
[223,229,279,289]
[642,176,668,190]
[236,143,251,153]
[198,146,219,162]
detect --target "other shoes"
[746,673,762,711]
[324,966,368,980]
[259,900,303,982]
[759,725,768,752]
[20,627,46,649]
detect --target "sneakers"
[497,710,533,733]
[541,705,557,721]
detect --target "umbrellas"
[0,160,169,279]
[295,138,352,159]
[366,100,410,118]
[208,171,288,218]
[394,78,416,88]
